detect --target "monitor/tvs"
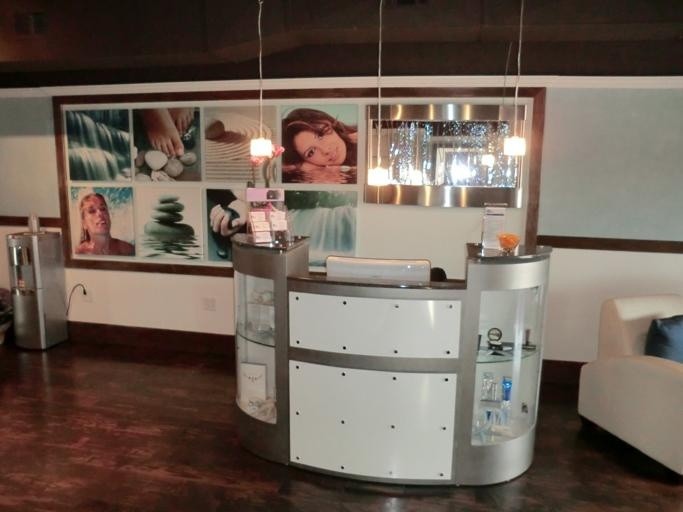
[326,255,431,282]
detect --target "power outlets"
[80,287,92,304]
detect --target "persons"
[282,108,358,170]
[136,108,196,156]
[206,189,267,236]
[73,193,135,256]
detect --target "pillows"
[645,313,683,363]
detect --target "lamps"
[502,0,527,156]
[249,2,274,158]
[367,1,391,187]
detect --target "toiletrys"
[500,376,513,406]
[28,209,40,233]
[481,372,493,401]
[489,379,498,401]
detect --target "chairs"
[577,292,683,480]
